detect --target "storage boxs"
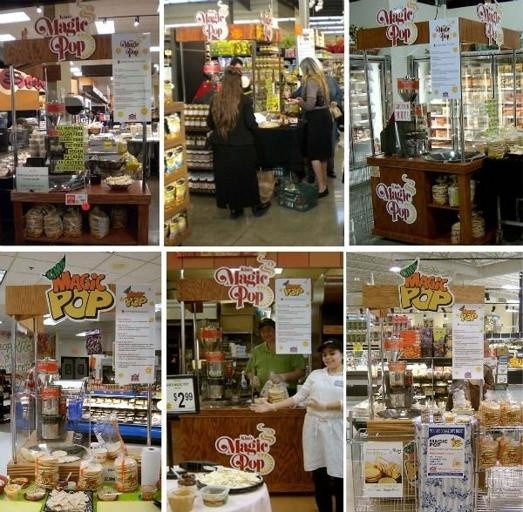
[220,302,257,315]
[219,311,259,333]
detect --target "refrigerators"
[405,45,522,154]
[347,54,395,190]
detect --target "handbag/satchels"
[277,181,318,212]
[264,168,286,177]
[256,170,275,205]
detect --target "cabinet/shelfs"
[366,148,494,245]
[164,101,192,247]
[350,49,522,185]
[185,125,217,195]
[219,329,261,400]
[9,177,152,246]
[76,388,162,443]
[345,311,523,397]
[203,36,344,119]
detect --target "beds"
[165,463,272,512]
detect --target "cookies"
[268,389,285,403]
[116,460,139,492]
[472,217,485,238]
[479,400,523,427]
[432,184,447,206]
[451,223,461,245]
[446,184,459,207]
[79,465,103,492]
[365,457,402,483]
[107,442,125,460]
[479,437,523,469]
[35,456,59,490]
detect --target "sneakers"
[230,209,243,219]
[318,185,329,197]
[253,202,271,217]
[328,168,335,179]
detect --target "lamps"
[102,14,140,28]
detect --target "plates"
[43,489,94,512]
[105,181,131,190]
[197,468,264,494]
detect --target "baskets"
[349,427,418,511]
[475,425,522,511]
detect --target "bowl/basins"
[167,487,197,512]
[199,483,230,507]
[89,173,102,184]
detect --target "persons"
[249,339,343,511]
[286,57,333,198]
[244,318,306,399]
[208,65,272,220]
[281,73,342,183]
[229,58,254,97]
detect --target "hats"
[259,318,274,328]
[317,338,343,352]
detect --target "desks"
[0,474,161,511]
